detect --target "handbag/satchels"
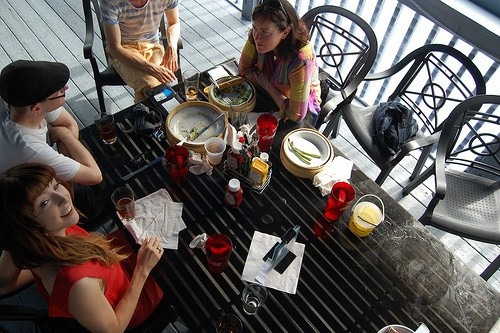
[369,101,419,160]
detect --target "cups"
[204,137,226,165]
[325,181,355,221]
[165,145,190,184]
[205,234,232,273]
[93,111,117,144]
[229,100,248,130]
[216,314,243,333]
[241,284,269,315]
[183,68,200,100]
[111,187,135,221]
[348,194,385,237]
[256,114,278,151]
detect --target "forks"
[207,76,232,104]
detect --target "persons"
[0,60,165,333]
[240,0,320,128]
[98,0,181,102]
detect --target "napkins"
[116,188,187,250]
[241,231,306,294]
[247,111,283,137]
[312,156,353,197]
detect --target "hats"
[0,59,70,108]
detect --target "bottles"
[226,142,245,173]
[249,152,272,187]
[223,179,244,210]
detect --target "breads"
[219,77,244,89]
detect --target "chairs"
[299,5,500,283]
[82,0,184,113]
[0,208,179,333]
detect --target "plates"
[376,325,416,333]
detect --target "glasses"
[258,0,291,25]
[45,86,66,100]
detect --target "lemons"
[204,86,209,94]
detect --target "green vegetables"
[213,81,251,105]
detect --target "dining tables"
[77,57,500,333]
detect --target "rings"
[157,247,163,253]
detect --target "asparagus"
[288,139,321,164]
